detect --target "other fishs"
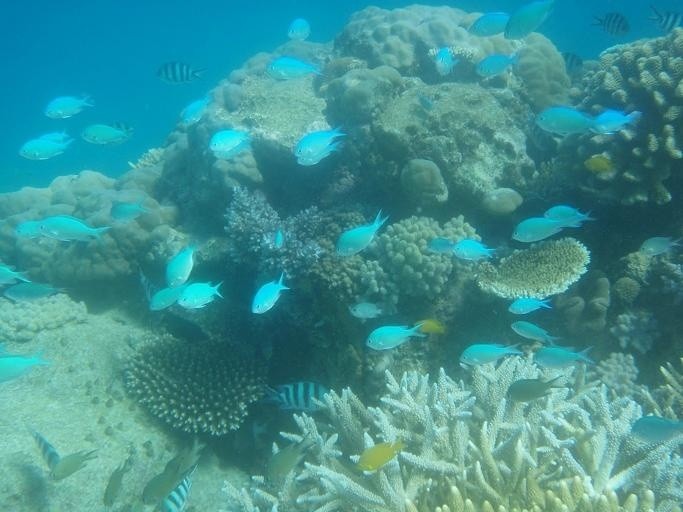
[0,0,683,413]
